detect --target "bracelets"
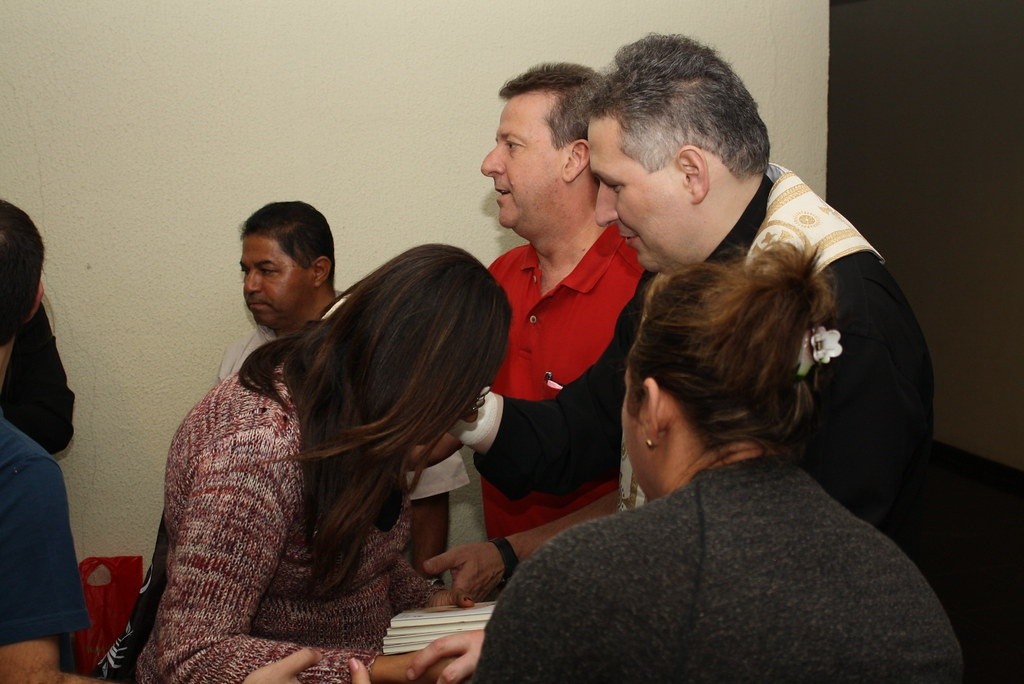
[428,579,444,585]
[490,537,519,589]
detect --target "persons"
[137,242,513,684]
[217,202,466,586]
[1,301,76,456]
[0,199,113,684]
[476,244,963,684]
[423,62,644,610]
[447,30,935,555]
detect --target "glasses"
[458,387,490,416]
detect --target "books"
[382,600,496,656]
